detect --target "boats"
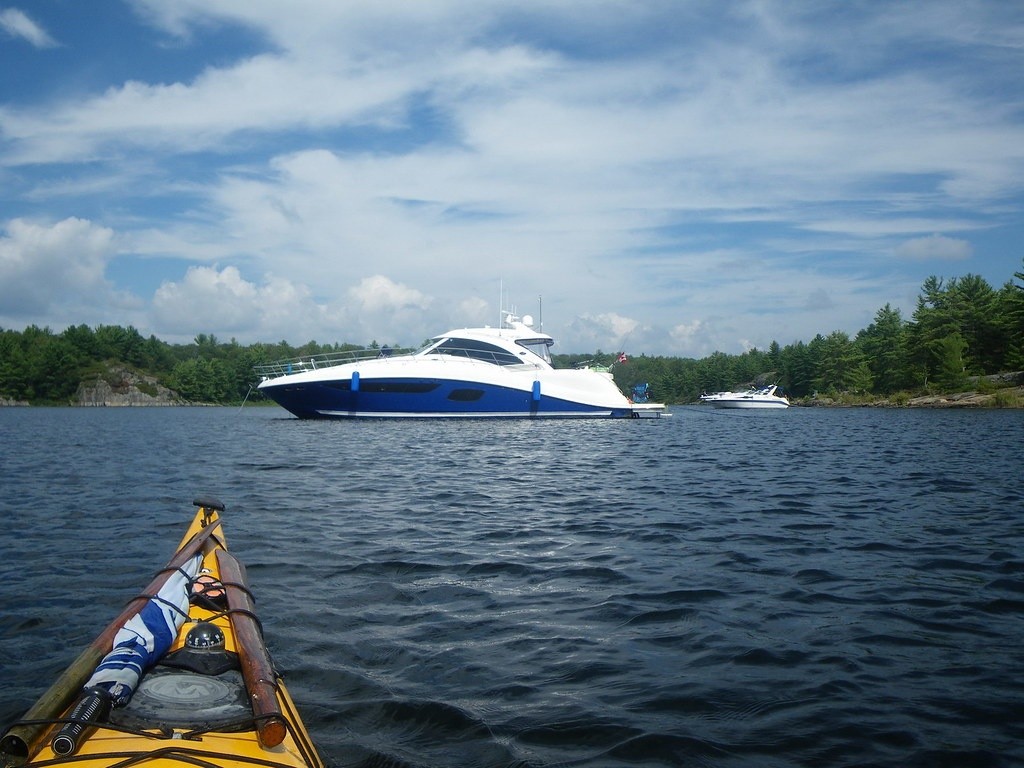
[258,278,636,419]
[2,496,321,768]
[701,385,791,410]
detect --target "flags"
[617,352,628,365]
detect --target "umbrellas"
[51,551,204,757]
